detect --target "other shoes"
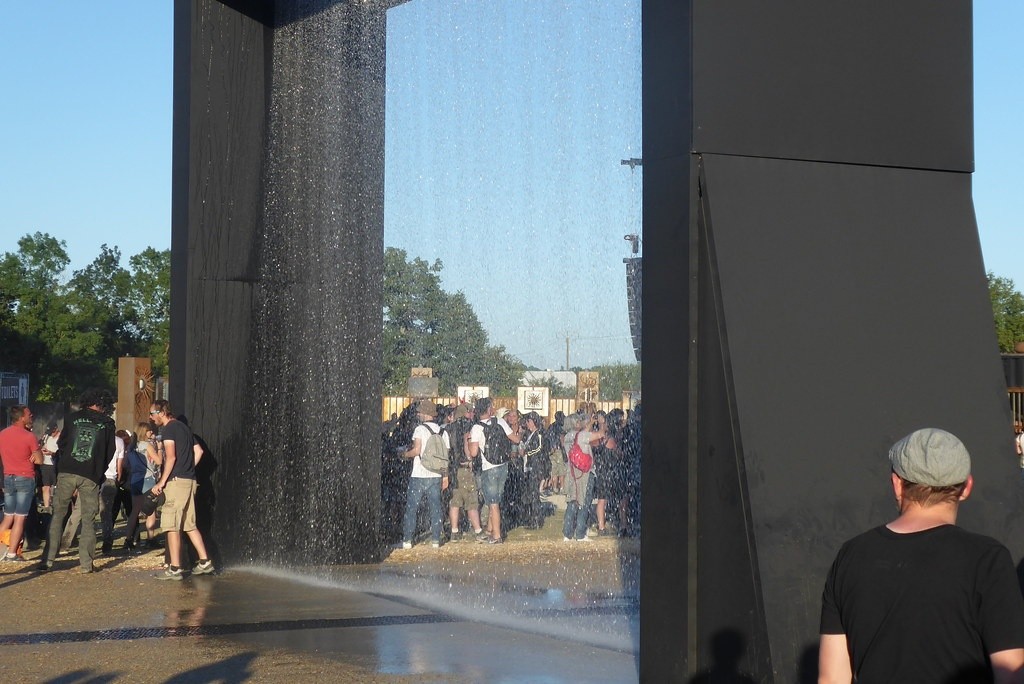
[103,537,161,556]
[476,530,491,542]
[450,531,463,542]
[37,561,52,571]
[618,527,629,538]
[485,536,503,544]
[403,541,412,549]
[586,527,598,537]
[4,554,25,561]
[81,565,100,573]
[564,536,575,541]
[597,528,611,536]
[577,535,592,541]
[434,541,440,549]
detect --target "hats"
[889,426,970,486]
[415,400,438,416]
[139,489,165,515]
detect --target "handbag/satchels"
[568,433,591,473]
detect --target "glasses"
[150,410,161,415]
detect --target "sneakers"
[153,564,183,580]
[191,560,214,575]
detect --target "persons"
[817,428,1024,684]
[148,400,215,581]
[383,396,642,548]
[0,404,43,562]
[22,421,167,554]
[37,389,117,573]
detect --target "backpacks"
[419,423,449,476]
[476,417,511,464]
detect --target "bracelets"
[158,449,161,450]
[467,438,471,441]
